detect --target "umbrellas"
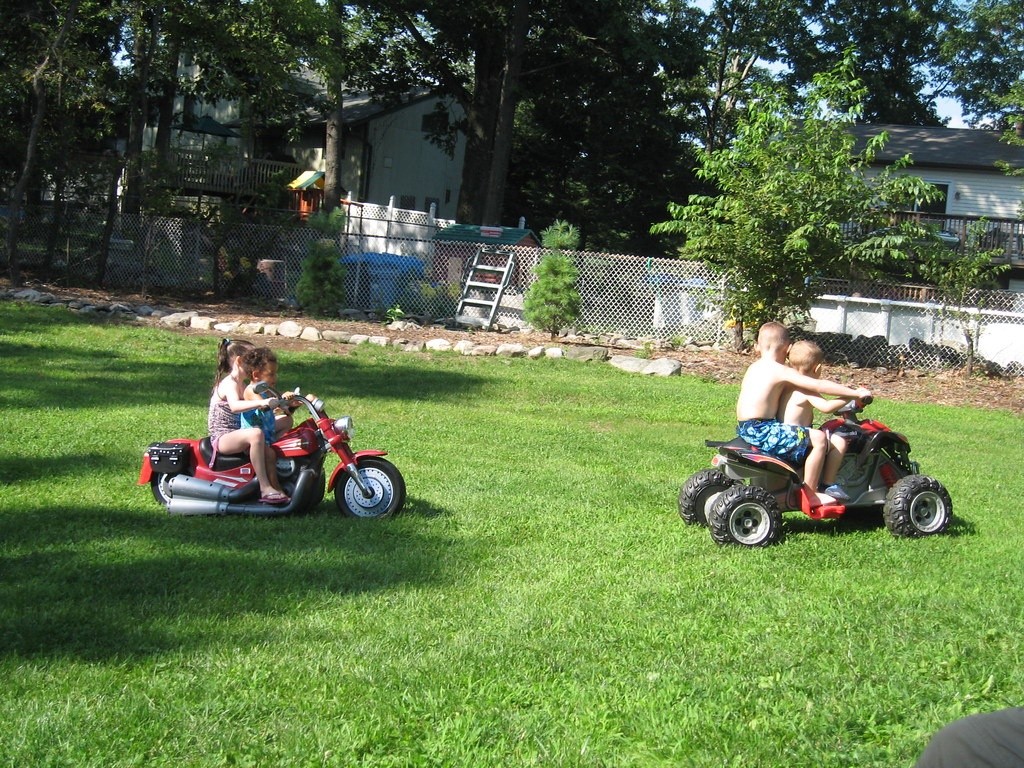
[223,118,251,128]
[169,113,242,151]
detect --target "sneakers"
[818,484,851,500]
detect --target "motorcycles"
[139,392,408,527]
[678,382,954,558]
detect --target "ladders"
[452,247,517,332]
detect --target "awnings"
[286,171,325,192]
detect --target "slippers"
[259,493,288,504]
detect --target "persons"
[208,338,303,505]
[736,322,874,506]
[241,347,303,503]
[776,341,853,500]
